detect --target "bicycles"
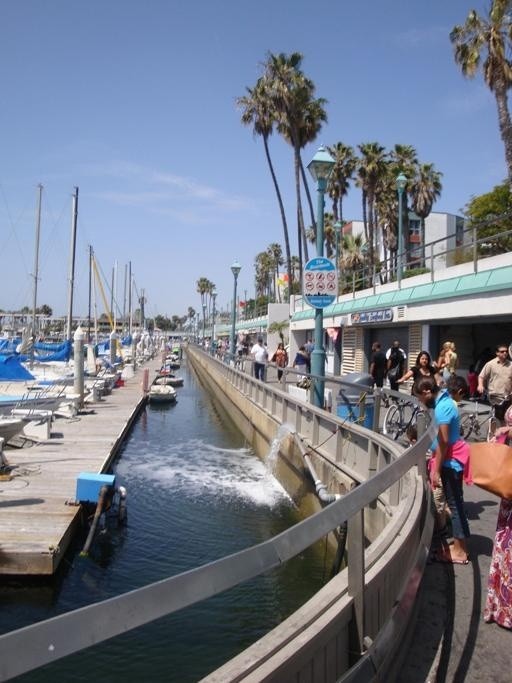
[460,392,511,444]
[384,380,431,440]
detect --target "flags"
[239,301,246,306]
[283,273,291,280]
[277,278,286,286]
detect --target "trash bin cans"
[336,372,375,430]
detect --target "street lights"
[307,142,325,409]
[333,221,342,267]
[394,172,406,291]
[228,257,241,366]
[185,289,218,356]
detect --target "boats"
[0,391,66,406]
[26,385,90,397]
[148,384,178,401]
[1,338,32,362]
[157,350,185,384]
[0,354,36,382]
[0,413,24,434]
[10,408,53,421]
[149,397,178,410]
[0,435,5,455]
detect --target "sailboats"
[0,180,153,391]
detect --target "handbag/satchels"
[388,367,400,380]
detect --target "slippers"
[430,545,470,564]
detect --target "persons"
[406,422,451,536]
[429,377,468,529]
[189,335,317,385]
[386,340,408,360]
[478,344,512,419]
[395,350,441,398]
[440,342,456,374]
[370,341,386,388]
[386,346,405,390]
[411,375,469,564]
[483,402,512,628]
